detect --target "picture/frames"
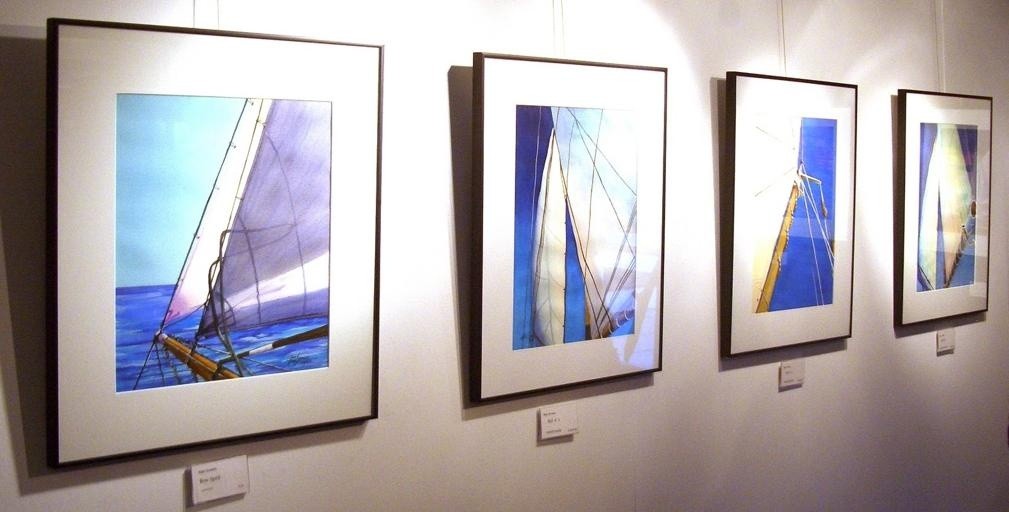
[465,45,672,412]
[45,14,388,473]
[891,82,996,339]
[718,67,862,361]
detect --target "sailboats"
[918,121,978,292]
[751,110,836,314]
[130,97,332,389]
[520,103,640,348]
